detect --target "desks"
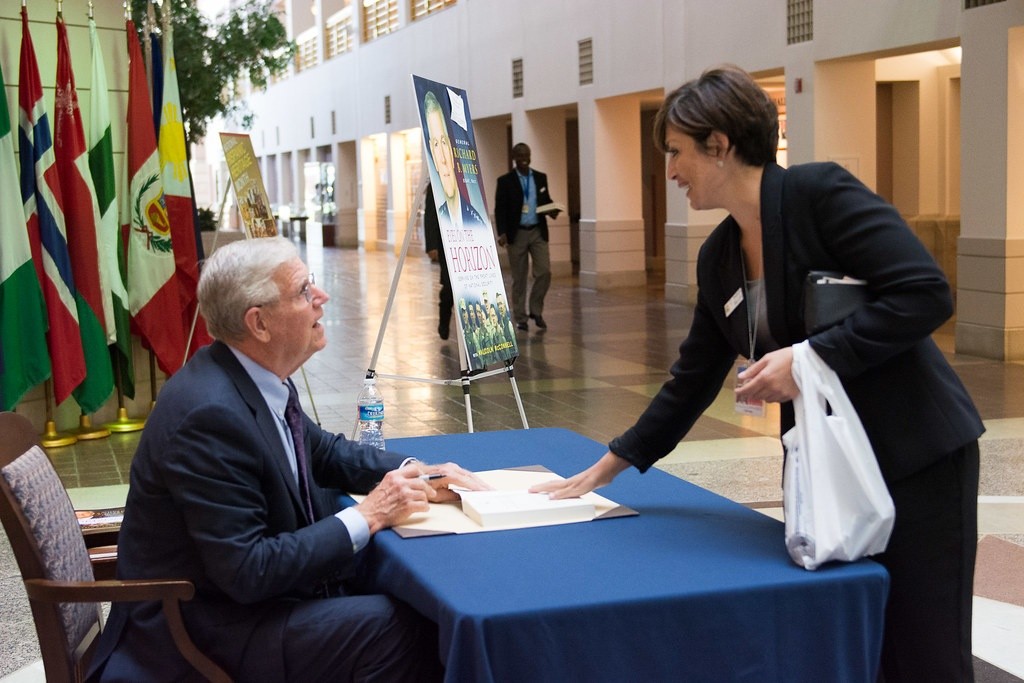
[339,424,890,683]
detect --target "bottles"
[357,379,385,452]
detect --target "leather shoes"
[529,313,547,328]
[516,321,528,331]
[438,322,450,339]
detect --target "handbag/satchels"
[781,340,896,573]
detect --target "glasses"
[243,271,316,323]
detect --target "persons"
[460,292,519,371]
[425,183,453,340]
[495,143,558,332]
[83,236,489,683]
[528,64,987,683]
[425,91,488,228]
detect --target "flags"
[0,5,215,415]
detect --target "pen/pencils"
[375,474,447,485]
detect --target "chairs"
[0,411,235,683]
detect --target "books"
[448,469,595,529]
[536,202,564,214]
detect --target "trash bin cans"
[322,224,335,247]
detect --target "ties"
[286,383,316,525]
